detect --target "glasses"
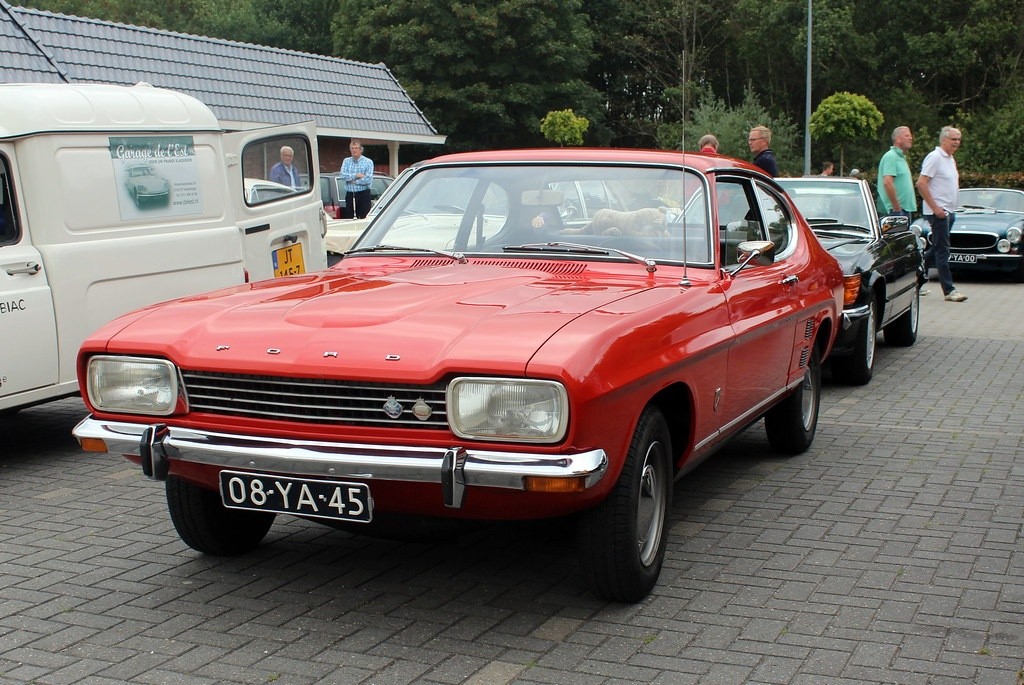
[747,138,764,142]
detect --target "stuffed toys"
[560,206,673,236]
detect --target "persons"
[340,141,374,219]
[749,126,781,178]
[850,169,859,180]
[821,162,834,176]
[918,127,968,302]
[685,135,739,208]
[269,146,302,192]
[878,126,917,230]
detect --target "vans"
[0,80,330,422]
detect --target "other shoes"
[919,287,932,296]
[943,289,968,302]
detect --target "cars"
[909,187,1024,283]
[70,146,847,606]
[241,169,630,271]
[669,172,928,386]
